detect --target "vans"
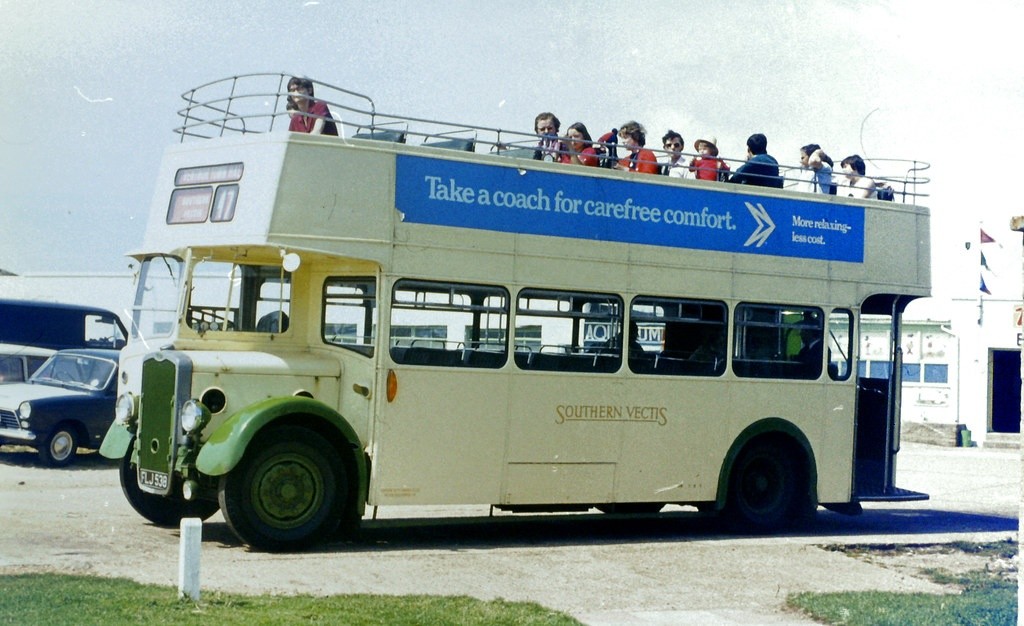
[1,291,153,401]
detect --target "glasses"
[537,127,552,131]
[664,143,681,147]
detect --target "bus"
[115,76,932,553]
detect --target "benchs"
[347,118,545,163]
[390,337,796,379]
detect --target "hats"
[694,137,718,156]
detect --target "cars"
[0,350,122,460]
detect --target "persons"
[794,320,831,380]
[613,122,658,174]
[836,155,877,198]
[797,144,837,195]
[287,77,337,136]
[565,123,599,167]
[661,131,697,179]
[534,113,572,164]
[689,137,730,182]
[620,322,644,357]
[598,129,619,161]
[728,134,783,189]
